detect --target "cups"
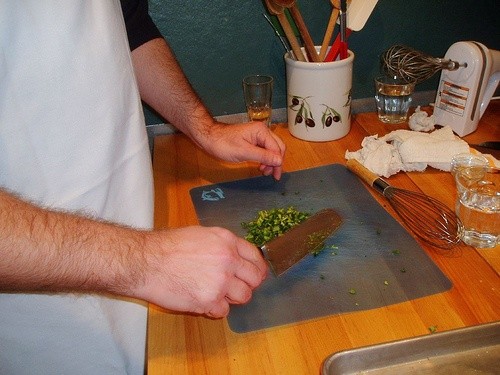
[284,45,354,142]
[376,77,414,123]
[456,165,500,249]
[244,74,272,129]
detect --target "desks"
[147,99,500,375]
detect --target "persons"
[0,0,286,375]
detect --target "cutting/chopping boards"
[190,164,453,333]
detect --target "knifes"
[258,209,344,278]
